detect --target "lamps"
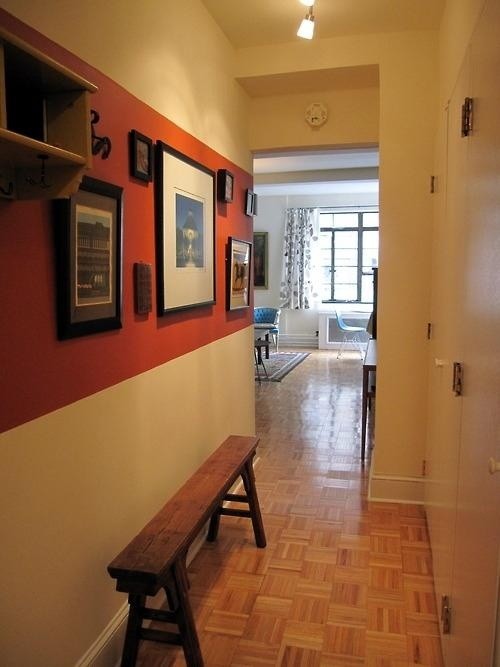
[297,0,315,40]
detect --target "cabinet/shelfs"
[0,27,97,177]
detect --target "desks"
[361,339,378,468]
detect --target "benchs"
[107,435,267,667]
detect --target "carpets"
[255,352,311,382]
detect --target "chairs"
[254,307,282,386]
[334,309,366,361]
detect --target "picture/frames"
[228,235,254,311]
[56,173,125,342]
[153,140,216,317]
[131,129,153,181]
[254,232,269,290]
[217,169,233,203]
[245,188,257,217]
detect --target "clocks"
[304,103,327,127]
